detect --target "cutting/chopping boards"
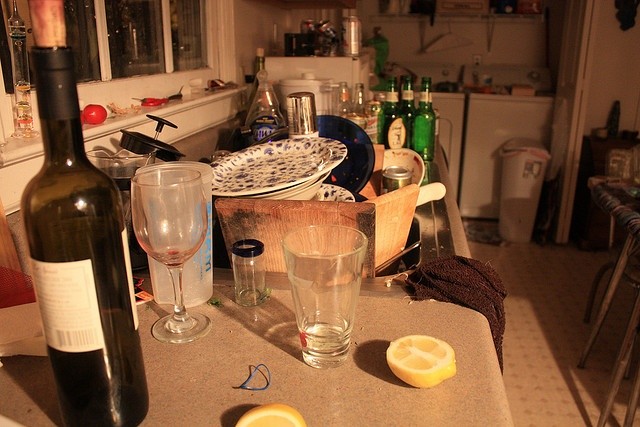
[360,183,420,276]
[214,198,376,277]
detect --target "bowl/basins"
[382,148,426,186]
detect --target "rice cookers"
[278,70,338,115]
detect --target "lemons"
[386,335,456,388]
[235,404,306,427]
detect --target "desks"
[577,177,640,427]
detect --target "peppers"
[132,97,170,103]
[141,98,169,106]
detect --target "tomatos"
[83,105,107,124]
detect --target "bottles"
[244,46,266,127]
[399,73,416,149]
[118,1,138,60]
[351,82,367,116]
[411,75,437,163]
[19,46,146,357]
[376,76,407,144]
[334,81,354,116]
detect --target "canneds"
[316,18,340,53]
[381,166,411,193]
[300,20,322,56]
[341,16,361,56]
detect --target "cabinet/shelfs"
[368,9,548,55]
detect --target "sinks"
[214,215,422,280]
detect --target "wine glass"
[131,167,213,344]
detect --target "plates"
[209,137,357,203]
[315,114,376,193]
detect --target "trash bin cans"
[497,138,550,243]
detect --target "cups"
[142,158,215,310]
[280,223,369,368]
[284,32,313,57]
[84,148,157,238]
